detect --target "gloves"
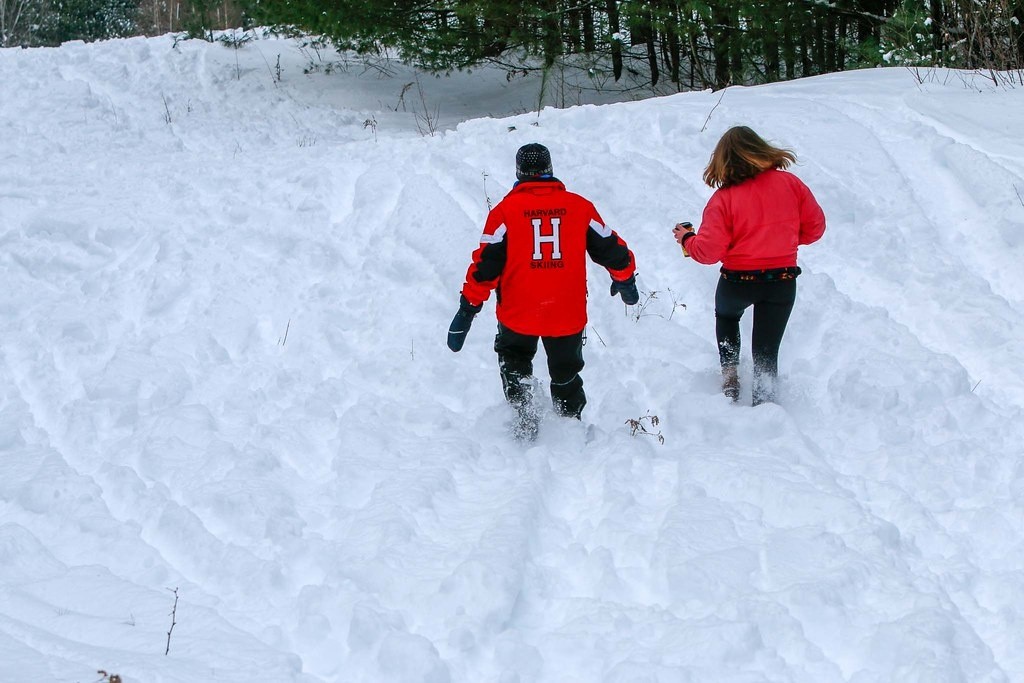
[447,306,476,352]
[610,273,639,305]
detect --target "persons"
[447,143,639,444]
[672,126,826,407]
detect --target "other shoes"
[513,419,538,444]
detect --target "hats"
[516,143,553,180]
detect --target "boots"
[722,367,740,401]
